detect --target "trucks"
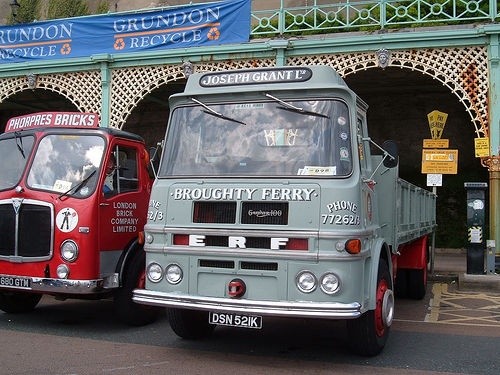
[131,61,440,360]
[1,110,164,328]
